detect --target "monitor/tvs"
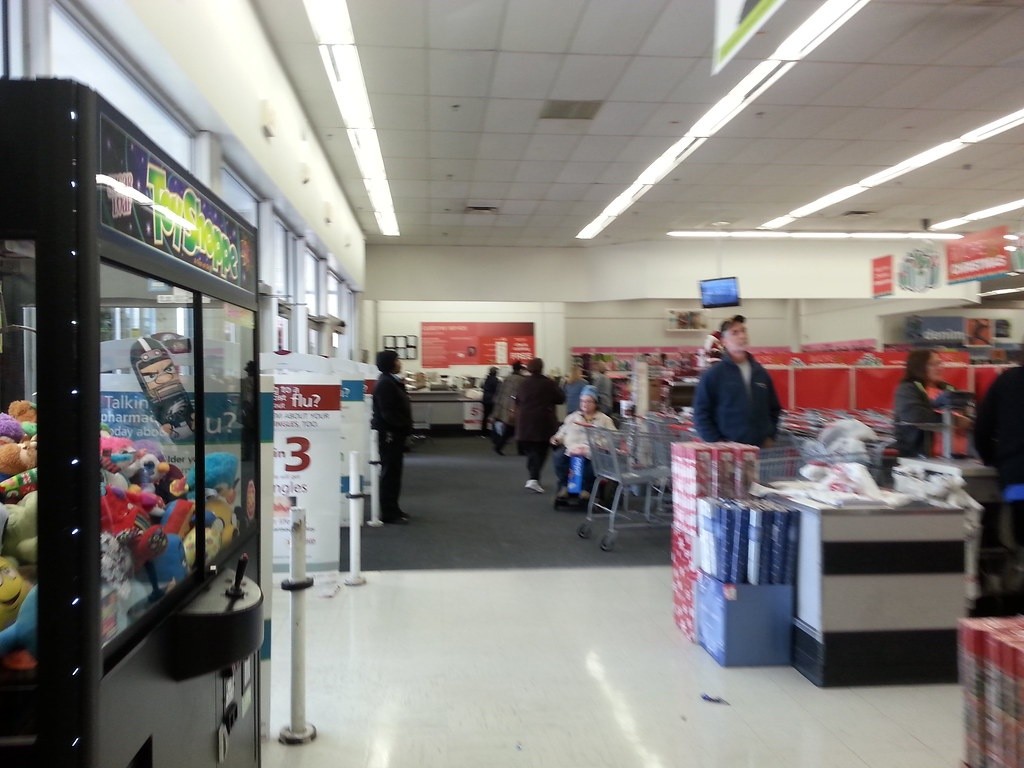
[669,386,695,407]
[700,277,740,308]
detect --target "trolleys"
[619,413,901,480]
[570,416,687,554]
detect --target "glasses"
[579,399,594,404]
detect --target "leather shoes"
[381,517,409,525]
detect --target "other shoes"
[493,445,506,456]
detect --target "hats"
[581,385,599,401]
[720,315,745,332]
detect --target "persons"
[372,351,413,524]
[970,367,1024,551]
[893,350,959,458]
[493,361,526,456]
[694,315,781,447]
[482,366,500,439]
[551,384,617,499]
[519,358,565,492]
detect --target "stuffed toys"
[0,400,241,672]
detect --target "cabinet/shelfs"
[772,484,982,689]
[407,386,484,431]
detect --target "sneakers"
[524,480,545,494]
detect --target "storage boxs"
[672,522,701,644]
[693,566,794,666]
